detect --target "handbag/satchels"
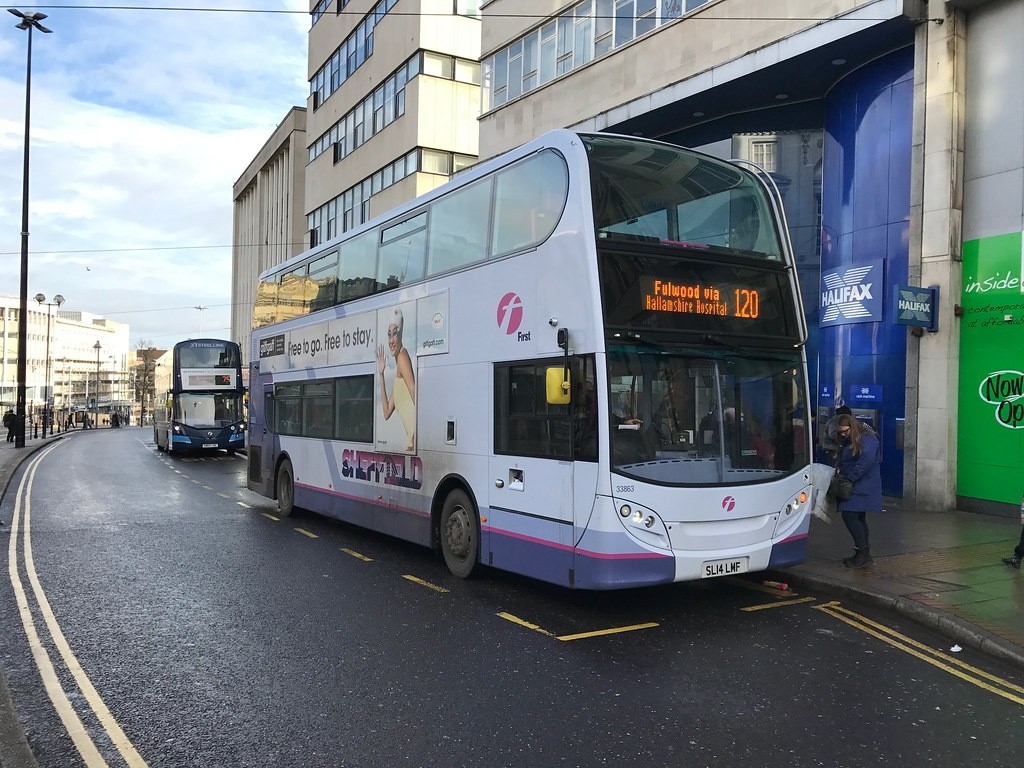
[834,474,853,500]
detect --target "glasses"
[838,426,851,434]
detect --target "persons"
[85,412,92,429]
[699,398,818,471]
[67,413,75,429]
[581,390,644,430]
[822,405,851,504]
[3,410,17,443]
[835,413,884,570]
[377,310,415,451]
[215,398,225,420]
[1002,491,1024,570]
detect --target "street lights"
[34,294,66,439]
[7,7,52,450]
[94,342,102,428]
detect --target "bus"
[246,129,814,591]
[153,338,249,456]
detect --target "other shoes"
[843,546,873,569]
[1002,554,1021,568]
[10,439,15,442]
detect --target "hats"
[389,309,403,331]
